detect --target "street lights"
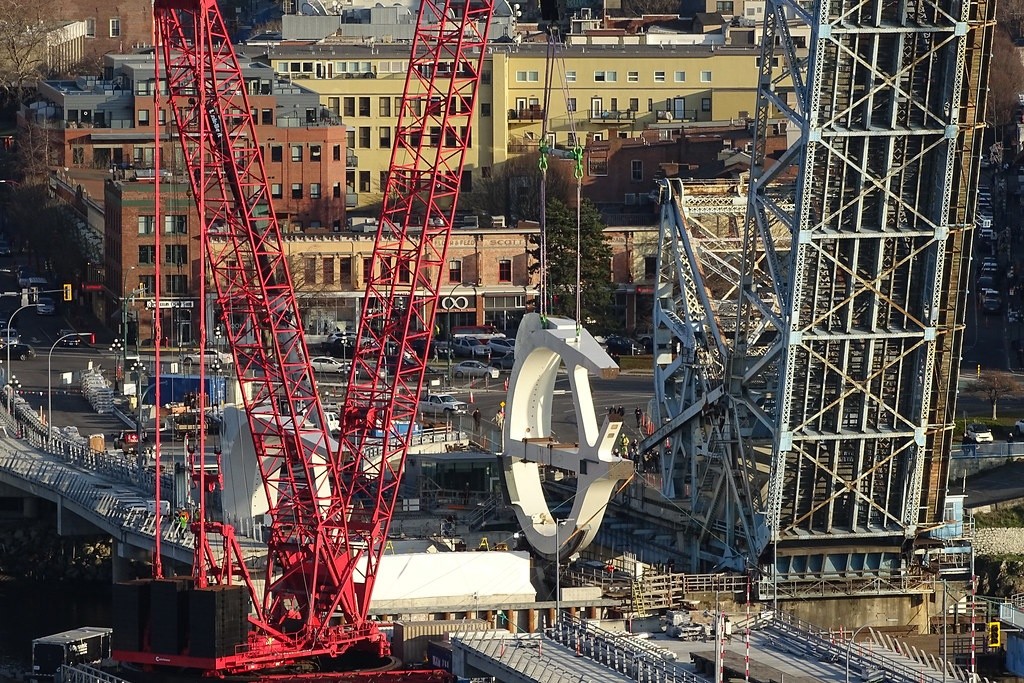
[7,303,46,415]
[212,361,222,427]
[759,509,777,618]
[131,363,147,492]
[920,580,947,683]
[846,618,898,683]
[49,332,92,442]
[144,307,155,374]
[108,340,124,393]
[448,282,477,387]
[556,518,574,632]
[8,375,23,422]
[216,326,221,363]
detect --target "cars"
[37,298,56,315]
[0,240,11,256]
[637,333,654,350]
[376,355,422,381]
[15,264,36,289]
[1015,418,1024,434]
[178,349,233,366]
[58,329,80,346]
[978,185,999,314]
[310,356,349,374]
[964,424,994,443]
[452,361,499,379]
[487,337,517,355]
[411,340,453,359]
[606,336,646,355]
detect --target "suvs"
[328,331,376,349]
[453,336,492,358]
[0,328,22,348]
[0,344,36,361]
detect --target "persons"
[608,404,617,414]
[635,406,642,428]
[495,409,503,427]
[174,507,189,540]
[617,404,625,429]
[472,408,481,432]
[612,432,660,473]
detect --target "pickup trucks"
[112,430,148,454]
[409,393,468,418]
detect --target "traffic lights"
[138,283,143,299]
[989,622,1001,647]
[64,285,71,301]
